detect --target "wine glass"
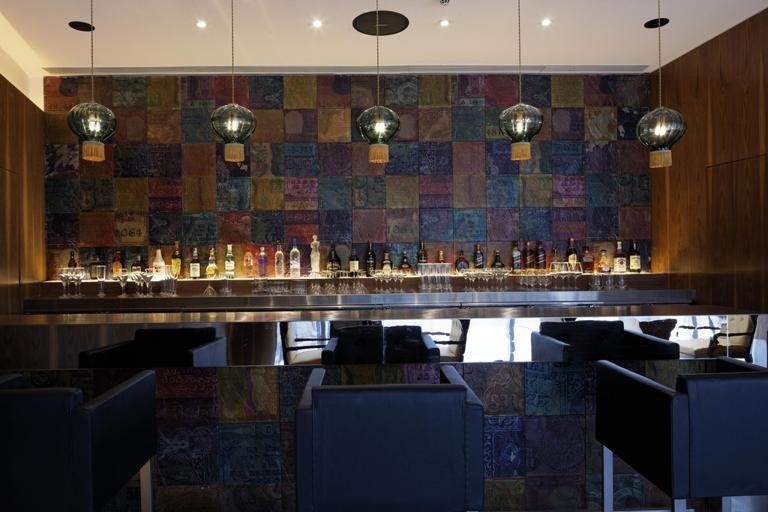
[59,261,631,293]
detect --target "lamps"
[213,0,256,162]
[66,0,115,161]
[357,0,399,164]
[637,0,685,168]
[500,0,541,161]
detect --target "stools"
[78,326,226,367]
[1,370,158,511]
[531,320,681,359]
[296,365,485,511]
[596,361,768,512]
[279,319,471,365]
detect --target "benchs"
[669,315,760,363]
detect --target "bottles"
[64,232,643,279]
